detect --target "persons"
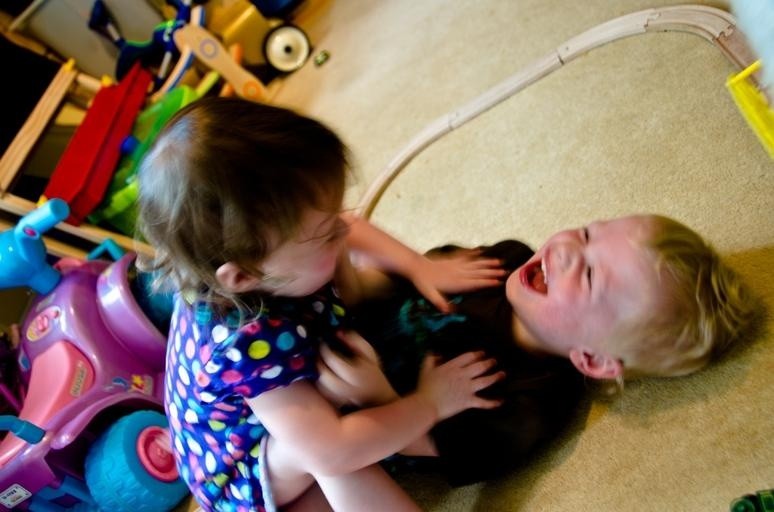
[226,214,765,511]
[136,98,505,511]
